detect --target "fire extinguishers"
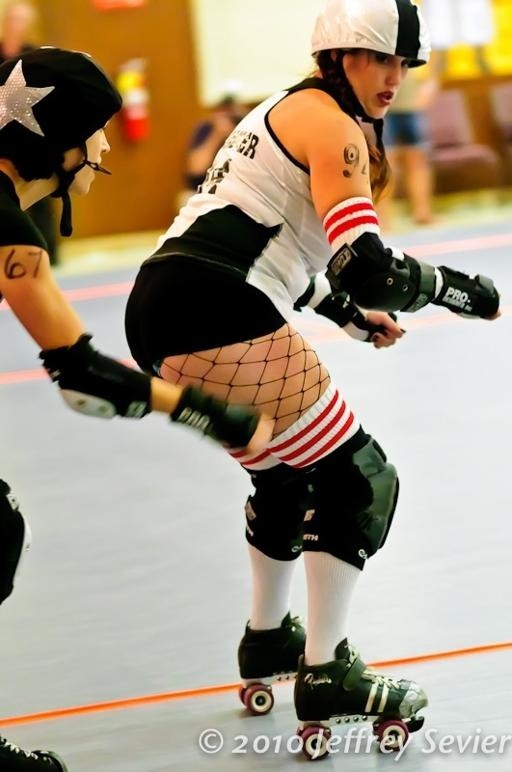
[114,56,151,139]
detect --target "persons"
[0,1,36,73]
[179,82,262,210]
[367,57,444,230]
[124,0,504,731]
[0,47,277,772]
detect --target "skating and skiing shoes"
[0,731,68,772]
[238,608,307,679]
[293,637,428,722]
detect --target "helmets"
[0,45,124,182]
[310,0,429,68]
